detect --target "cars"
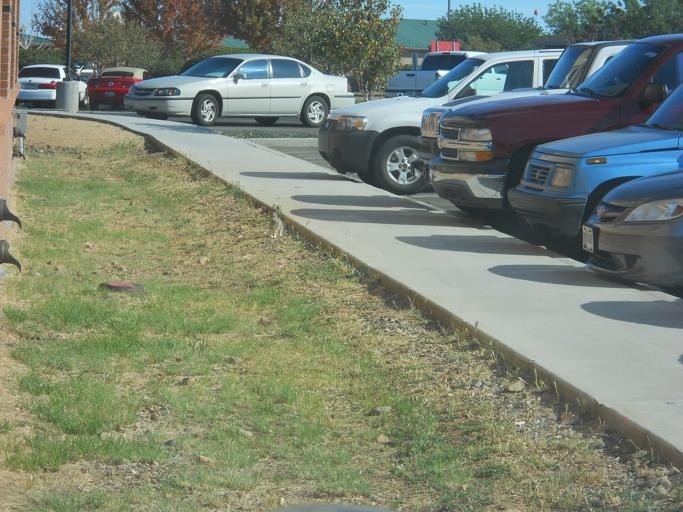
[85,66,153,111]
[14,63,97,111]
[124,53,355,129]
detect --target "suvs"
[315,42,567,195]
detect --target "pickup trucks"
[381,49,511,98]
